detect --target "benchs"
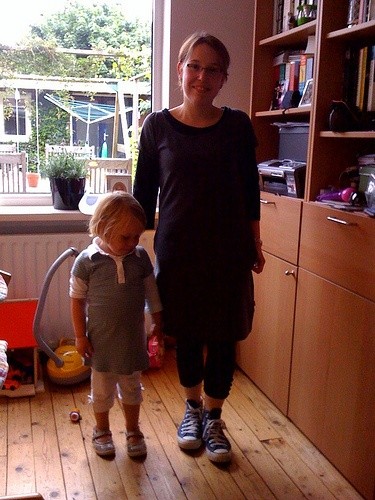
[46,144,96,160]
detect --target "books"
[343,45,375,113]
[271,50,313,99]
[270,0,316,36]
[347,0,375,28]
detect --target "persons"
[132,33,267,462]
[69,190,164,458]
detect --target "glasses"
[184,63,223,75]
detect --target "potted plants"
[28,150,39,188]
[41,145,89,210]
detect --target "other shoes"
[124,430,148,457]
[92,425,124,460]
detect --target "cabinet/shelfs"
[234,0,375,500]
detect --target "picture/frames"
[106,175,133,195]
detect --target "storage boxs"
[279,128,310,163]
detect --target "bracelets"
[249,238,264,246]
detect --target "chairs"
[88,159,133,194]
[0,143,16,153]
[0,152,27,194]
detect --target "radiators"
[0,230,159,347]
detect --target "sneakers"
[177,403,203,450]
[203,418,233,463]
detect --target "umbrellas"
[45,95,138,145]
